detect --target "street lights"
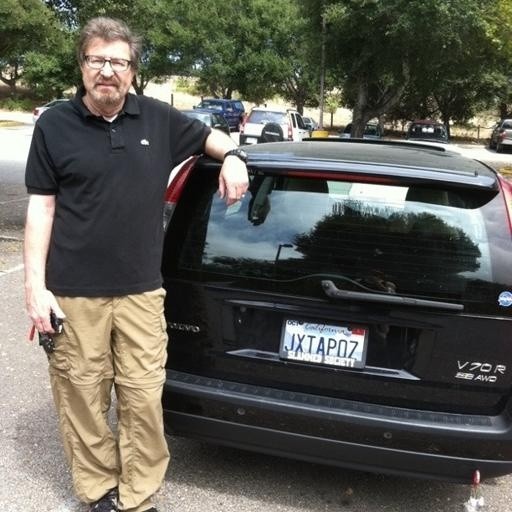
[276,242,295,262]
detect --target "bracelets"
[224,149,248,164]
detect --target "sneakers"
[92,488,119,512]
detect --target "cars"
[176,107,232,141]
[338,119,385,143]
[489,117,512,154]
[31,98,75,124]
[405,120,449,143]
[302,113,319,130]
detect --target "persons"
[24,16,252,512]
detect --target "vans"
[160,133,512,489]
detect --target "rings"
[241,194,245,198]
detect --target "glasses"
[84,55,132,72]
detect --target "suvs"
[192,97,248,132]
[237,103,313,148]
